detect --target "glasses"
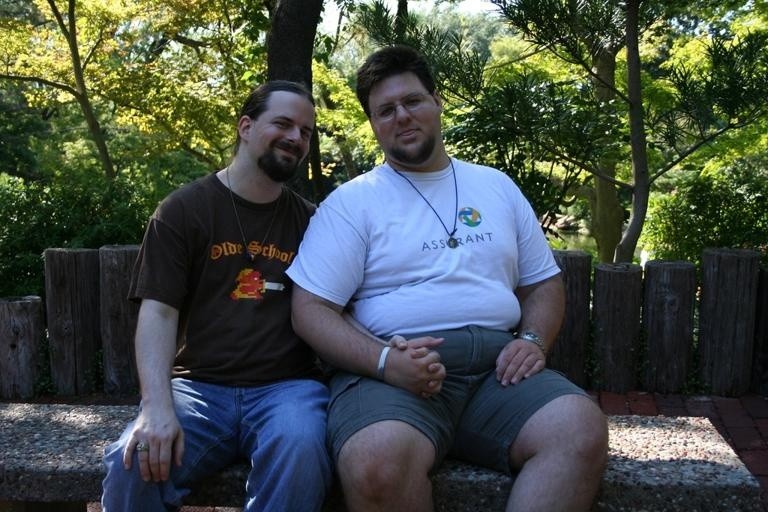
[366,91,432,120]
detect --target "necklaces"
[391,157,461,250]
[223,163,285,261]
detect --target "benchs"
[1,403,762,510]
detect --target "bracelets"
[376,344,394,384]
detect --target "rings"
[134,441,150,453]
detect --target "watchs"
[516,329,546,357]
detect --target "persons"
[101,79,337,511]
[283,46,610,512]
